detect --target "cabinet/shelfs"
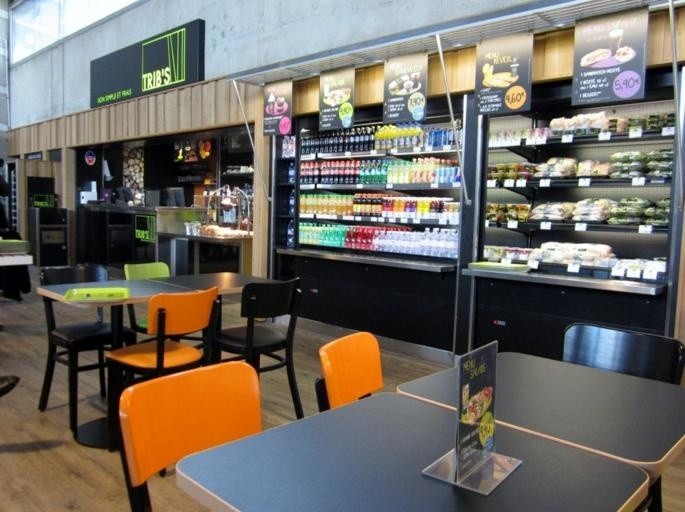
[460,85,685,364]
[270,112,465,365]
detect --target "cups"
[192,223,201,237]
[273,97,286,115]
[609,27,624,57]
[186,226,192,235]
[409,70,423,91]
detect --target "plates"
[393,84,422,98]
[262,102,289,118]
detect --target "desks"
[149,271,308,365]
[172,389,653,512]
[393,350,684,512]
[33,268,195,455]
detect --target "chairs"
[122,261,183,343]
[562,321,685,512]
[36,262,139,432]
[103,284,225,478]
[116,359,265,512]
[219,273,312,418]
[311,329,388,413]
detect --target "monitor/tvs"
[167,186,186,207]
[80,191,98,205]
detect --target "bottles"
[608,109,618,133]
[287,122,464,259]
